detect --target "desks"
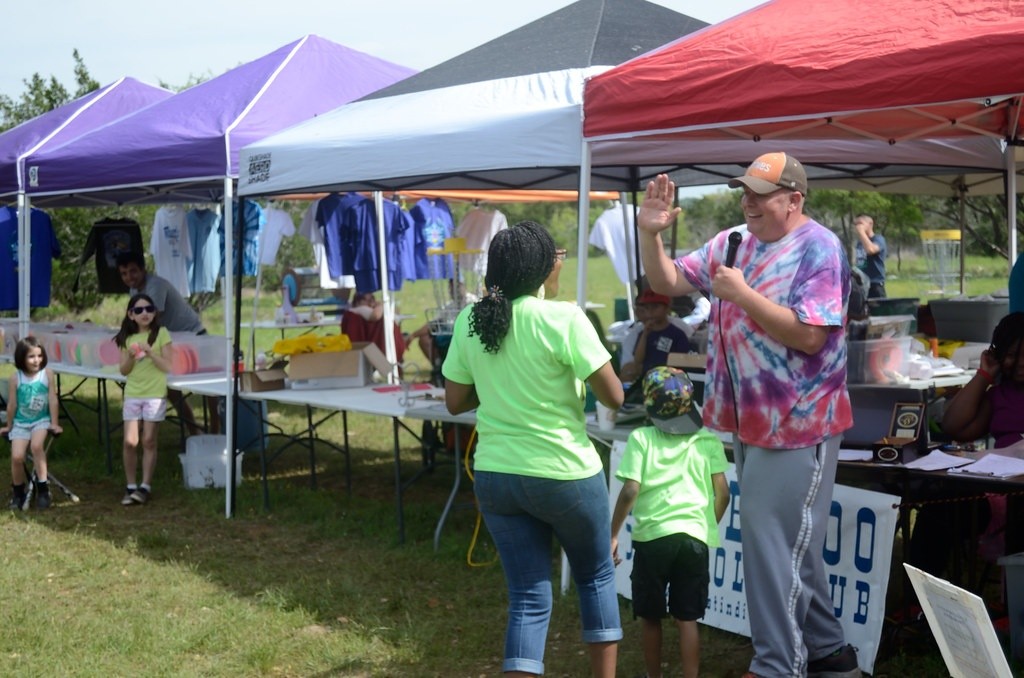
[0,302,1024,659]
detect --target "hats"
[639,289,670,305]
[728,152,807,196]
[642,366,704,435]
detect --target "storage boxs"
[240,370,290,393]
[288,343,395,390]
[848,336,913,381]
[668,352,707,382]
[178,435,244,488]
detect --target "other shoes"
[36,481,50,507]
[131,488,149,504]
[10,484,26,509]
[121,489,135,504]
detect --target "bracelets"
[976,368,993,385]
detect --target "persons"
[113,293,172,505]
[405,280,481,365]
[893,311,1024,630]
[610,367,730,678]
[0,336,63,509]
[342,291,383,344]
[117,249,221,438]
[638,152,860,678]
[442,221,624,678]
[851,214,887,300]
[616,286,712,401]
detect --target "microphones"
[725,232,742,268]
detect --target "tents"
[578,0,1024,306]
[230,2,1024,523]
[0,36,416,519]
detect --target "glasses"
[131,305,154,314]
[555,249,567,260]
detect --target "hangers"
[7,201,54,220]
[91,205,138,226]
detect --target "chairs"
[0,394,81,511]
